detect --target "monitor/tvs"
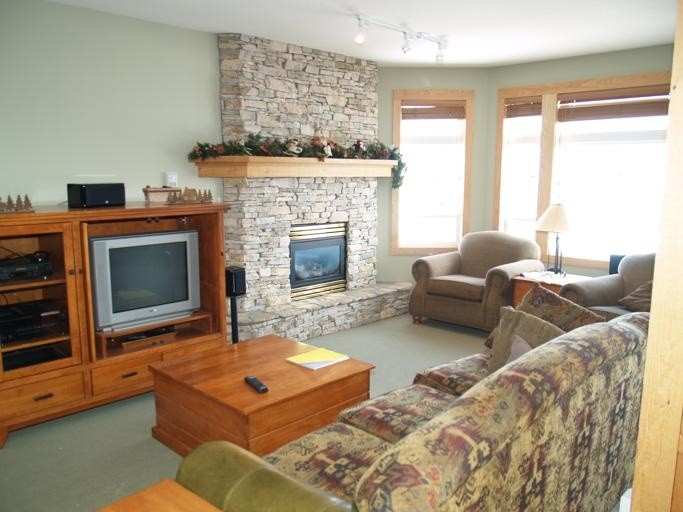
[88,229,201,333]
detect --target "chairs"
[556,251,655,321]
[408,231,550,333]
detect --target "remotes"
[244,376,268,394]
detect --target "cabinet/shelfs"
[0,202,89,449]
[510,271,595,310]
[75,200,234,409]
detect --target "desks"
[95,478,225,512]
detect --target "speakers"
[226,266,246,297]
[67,183,126,208]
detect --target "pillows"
[486,303,569,375]
[615,278,653,315]
[481,279,606,350]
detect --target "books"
[286,347,349,371]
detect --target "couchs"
[172,293,649,512]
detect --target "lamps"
[532,202,570,279]
[355,13,450,63]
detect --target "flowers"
[185,131,405,189]
